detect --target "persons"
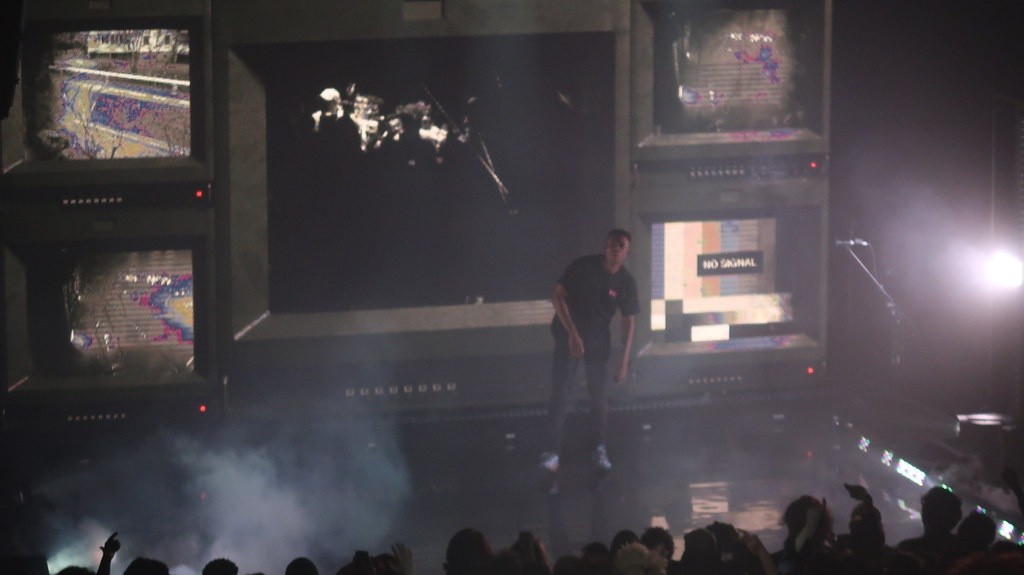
[535,228,641,475]
[1,463,1024,574]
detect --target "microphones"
[837,239,868,246]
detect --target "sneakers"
[591,446,612,472]
[538,452,559,474]
